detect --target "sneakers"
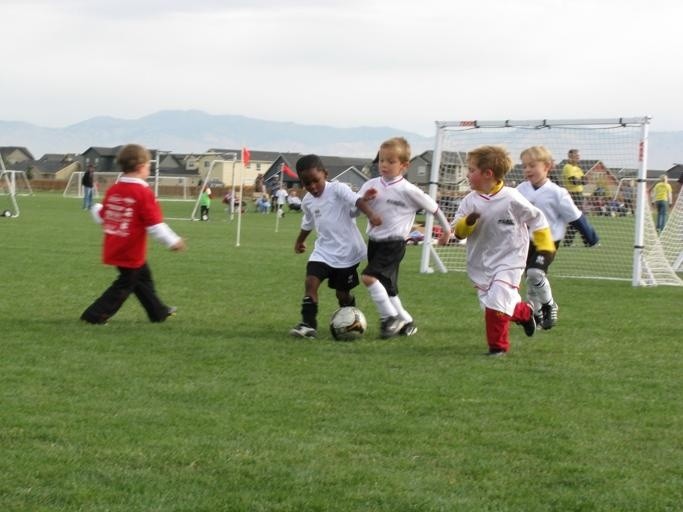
[379,313,417,338]
[525,298,559,336]
[167,304,176,315]
[290,323,318,338]
[486,347,505,357]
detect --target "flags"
[243,147,250,166]
[280,165,298,178]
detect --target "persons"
[198,174,302,221]
[289,153,384,338]
[453,144,558,356]
[649,174,673,233]
[80,142,185,324]
[561,148,600,248]
[350,136,452,339]
[80,163,94,211]
[513,145,602,331]
[621,180,635,215]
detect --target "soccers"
[329,306,367,340]
[202,215,208,221]
[4,209,12,217]
